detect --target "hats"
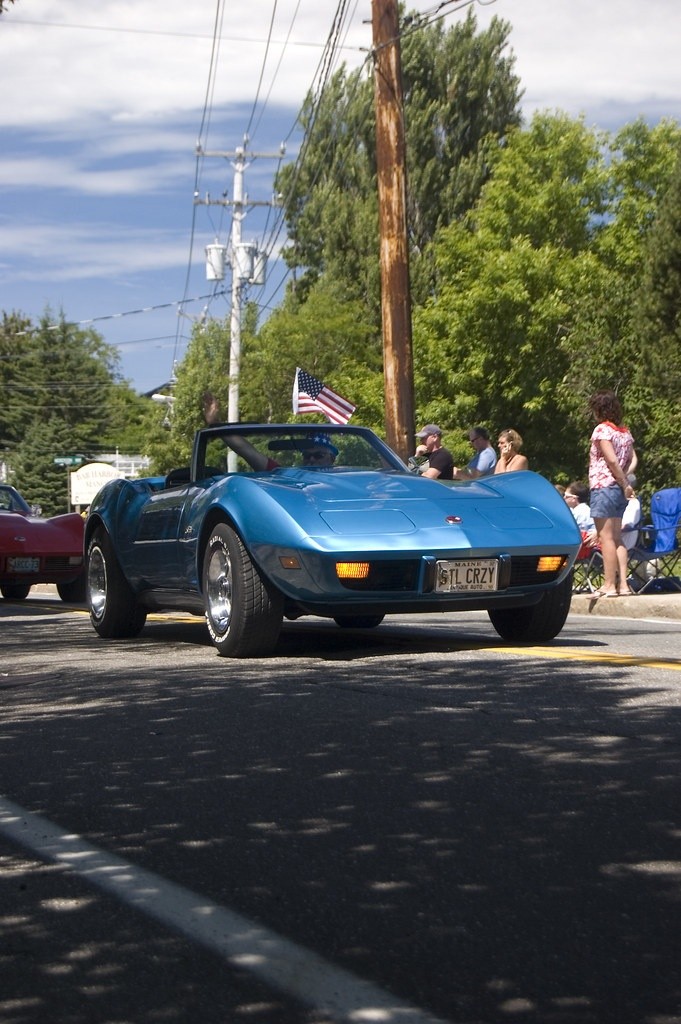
[299,432,339,456]
[621,498,642,530]
[413,424,442,438]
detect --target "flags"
[291,366,358,425]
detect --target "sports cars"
[0,486,86,603]
[81,423,585,658]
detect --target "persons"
[415,424,641,564]
[584,388,638,600]
[198,392,339,473]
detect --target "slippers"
[617,591,633,596]
[585,589,618,599]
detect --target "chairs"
[575,488,681,592]
[165,464,224,491]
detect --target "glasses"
[303,452,333,460]
[507,430,512,433]
[471,436,480,442]
[564,492,579,499]
